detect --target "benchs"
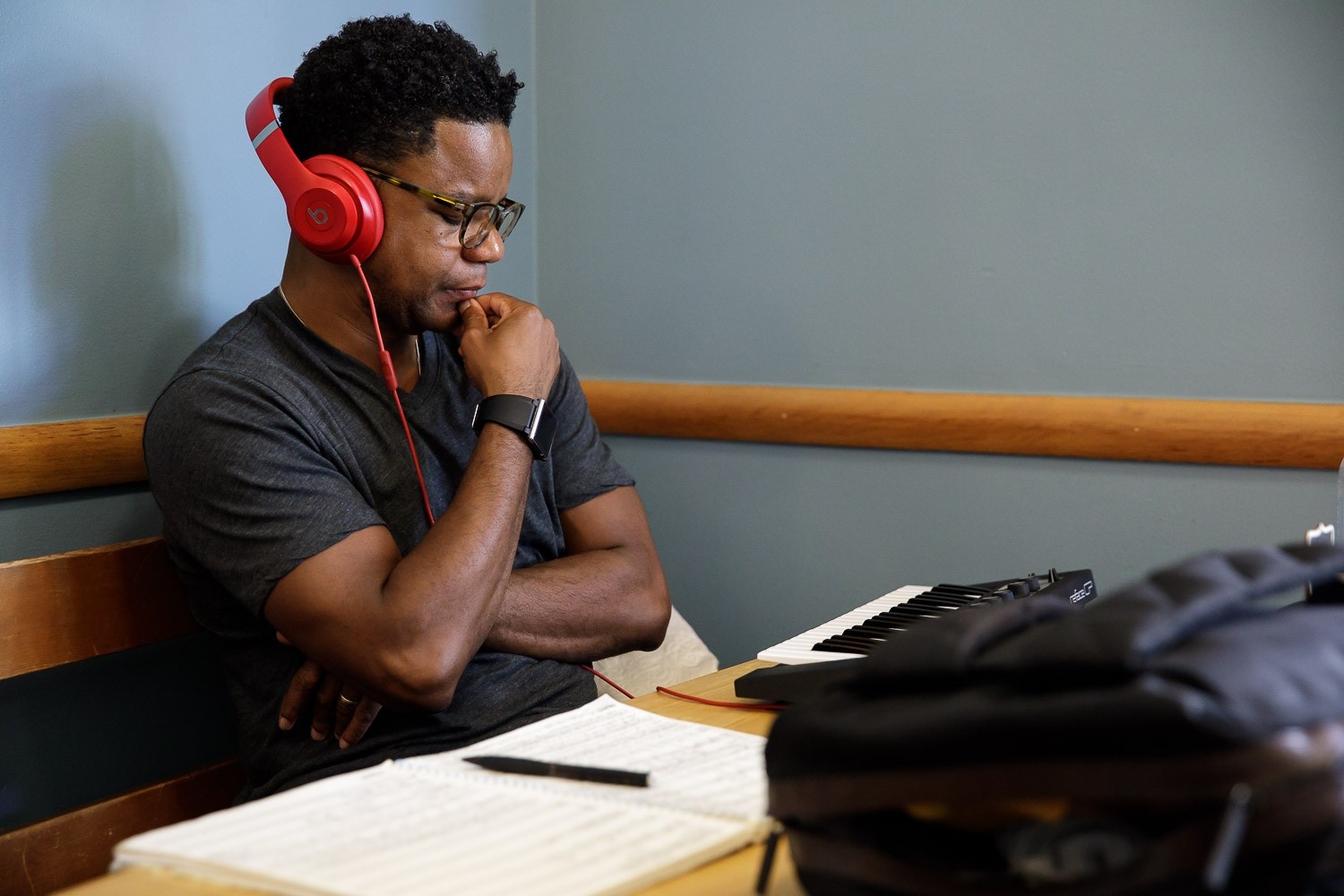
[0,414,248,896]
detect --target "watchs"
[471,393,559,463]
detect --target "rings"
[339,695,355,704]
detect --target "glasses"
[360,166,525,249]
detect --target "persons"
[143,15,671,800]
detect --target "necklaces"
[279,283,421,375]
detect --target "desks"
[46,654,1065,896]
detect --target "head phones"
[245,77,386,268]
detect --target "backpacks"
[765,524,1344,895]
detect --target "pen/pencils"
[463,752,648,787]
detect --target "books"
[108,694,788,895]
